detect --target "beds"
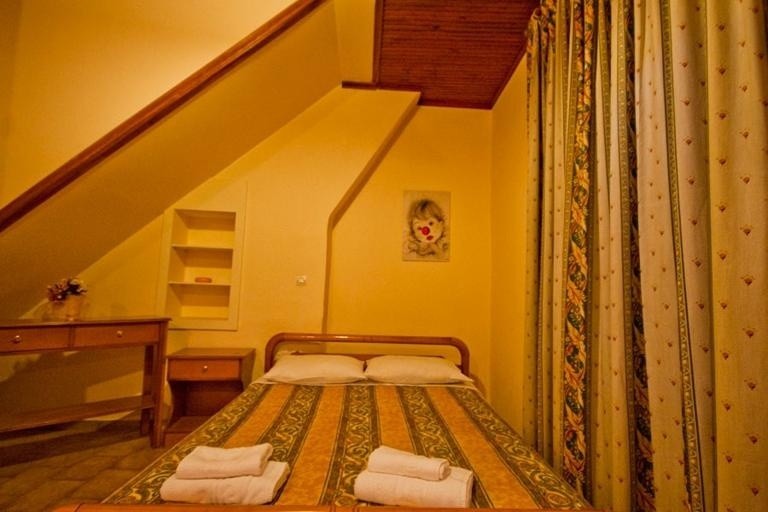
[49,332,609,511]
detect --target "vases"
[50,301,63,321]
[65,295,83,321]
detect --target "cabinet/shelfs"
[152,197,247,331]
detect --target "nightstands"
[163,347,255,448]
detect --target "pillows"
[263,353,368,386]
[364,355,474,385]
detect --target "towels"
[354,465,473,508]
[175,442,274,479]
[159,462,291,506]
[367,445,451,480]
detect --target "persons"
[403,198,449,259]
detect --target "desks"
[1,314,172,446]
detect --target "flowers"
[46,275,67,301]
[67,278,88,294]
